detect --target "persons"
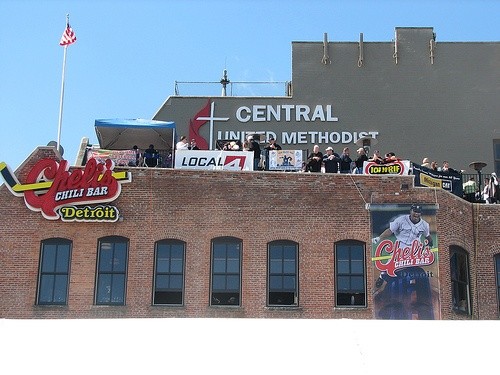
[421,158,500,204]
[131,144,160,167]
[176,135,200,150]
[301,144,399,174]
[371,203,433,287]
[222,135,282,170]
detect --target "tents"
[95,118,175,169]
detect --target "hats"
[248,136,254,140]
[268,137,275,142]
[411,203,424,213]
[326,146,334,151]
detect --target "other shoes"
[375,277,384,288]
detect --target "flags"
[59,21,76,46]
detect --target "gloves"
[372,236,380,244]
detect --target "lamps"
[400,184,408,190]
